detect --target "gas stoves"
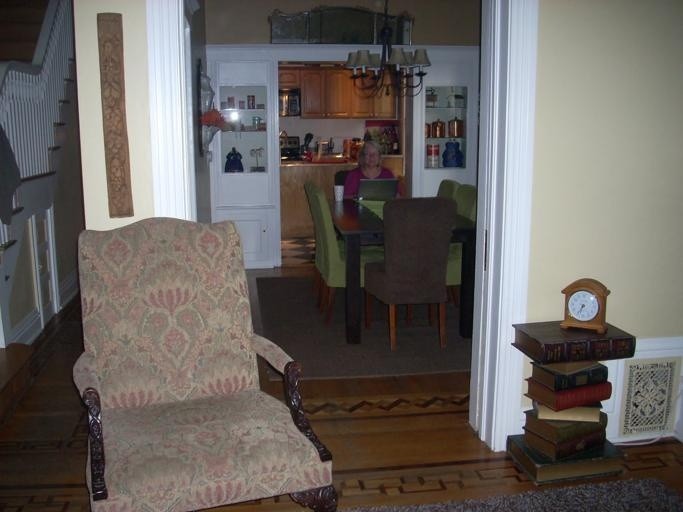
[281,148,300,161]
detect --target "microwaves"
[279,87,301,117]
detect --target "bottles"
[431,116,445,138]
[448,116,464,138]
[220,95,255,109]
[252,117,260,129]
[342,137,352,158]
[383,126,399,155]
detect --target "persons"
[342,139,405,201]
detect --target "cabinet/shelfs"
[404,46,478,200]
[300,69,352,118]
[207,46,277,270]
[351,69,396,119]
[277,69,300,90]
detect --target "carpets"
[341,476,683,512]
[255,275,473,382]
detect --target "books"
[509,319,637,365]
[522,377,612,412]
[530,398,602,423]
[505,434,626,486]
[528,359,609,391]
[522,408,607,443]
[521,426,606,459]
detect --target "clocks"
[559,278,611,334]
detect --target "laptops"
[357,179,398,200]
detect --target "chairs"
[302,169,478,353]
[70,216,338,512]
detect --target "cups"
[334,185,345,202]
[427,144,440,168]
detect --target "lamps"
[343,0,433,99]
